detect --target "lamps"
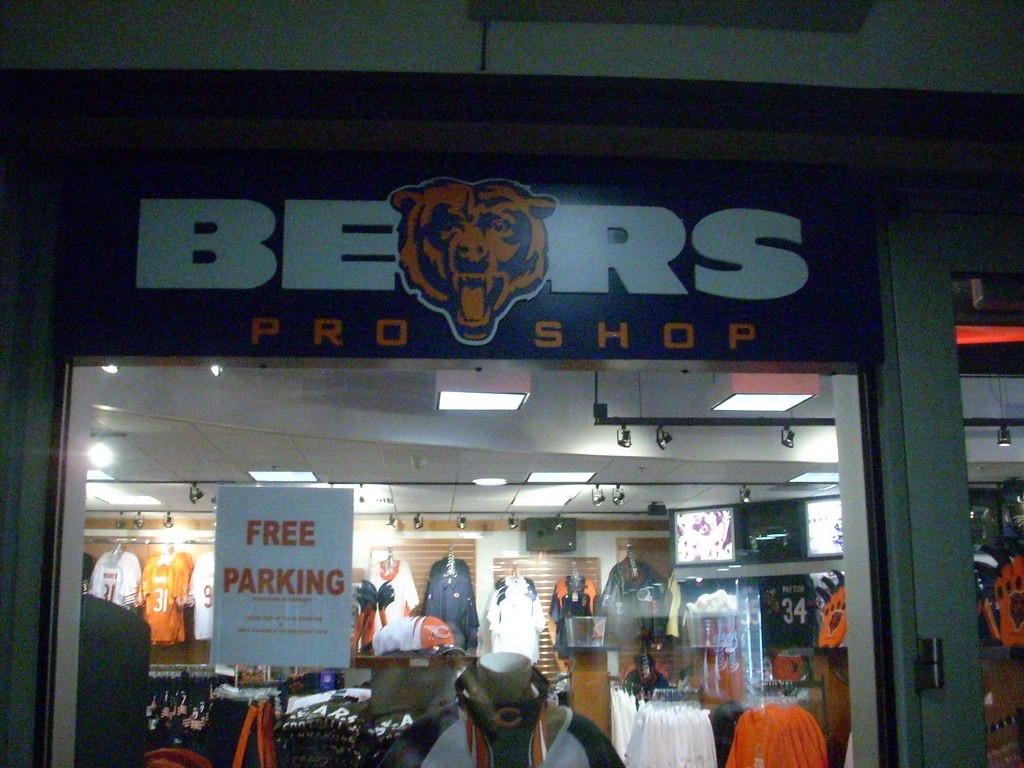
[189,486,203,504]
[386,515,395,529]
[997,426,1011,446]
[612,488,625,506]
[413,514,424,529]
[591,488,605,507]
[616,429,632,448]
[656,429,673,450]
[739,488,752,505]
[507,514,519,529]
[781,425,795,448]
[133,512,144,530]
[162,512,173,529]
[457,515,467,529]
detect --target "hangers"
[149,663,213,679]
[104,542,125,568]
[444,547,457,579]
[214,671,282,713]
[616,679,644,700]
[156,544,175,567]
[644,686,703,712]
[383,545,395,575]
[753,679,798,714]
[622,548,641,580]
[566,566,589,597]
